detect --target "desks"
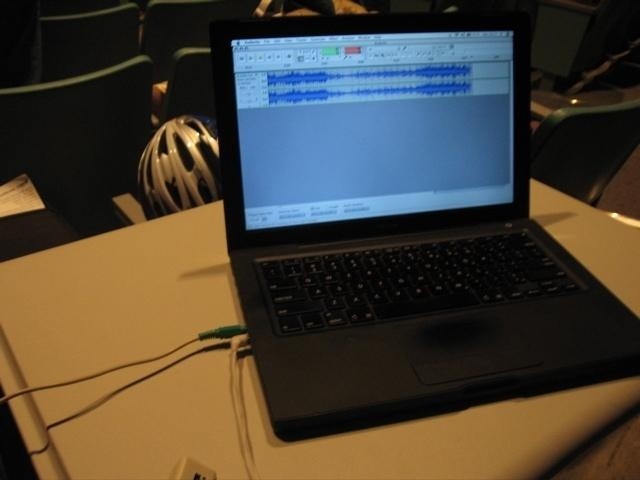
[0,177,640,480]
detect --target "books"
[0,174,47,219]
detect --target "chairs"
[31,4,140,84]
[1,54,152,177]
[141,1,261,60]
[528,99,639,208]
[159,47,219,127]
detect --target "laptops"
[207,10,640,443]
